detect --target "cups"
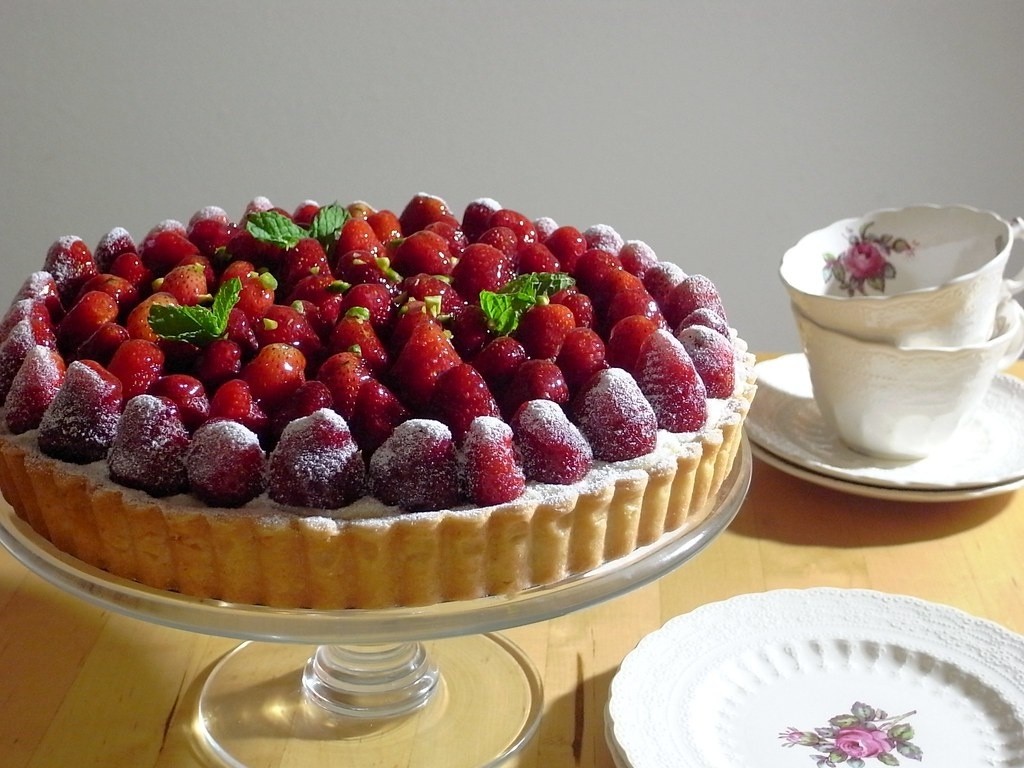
[791,299,1022,460]
[779,204,1024,348]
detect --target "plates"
[744,352,1024,503]
[603,586,1024,768]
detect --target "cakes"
[0,193,760,611]
